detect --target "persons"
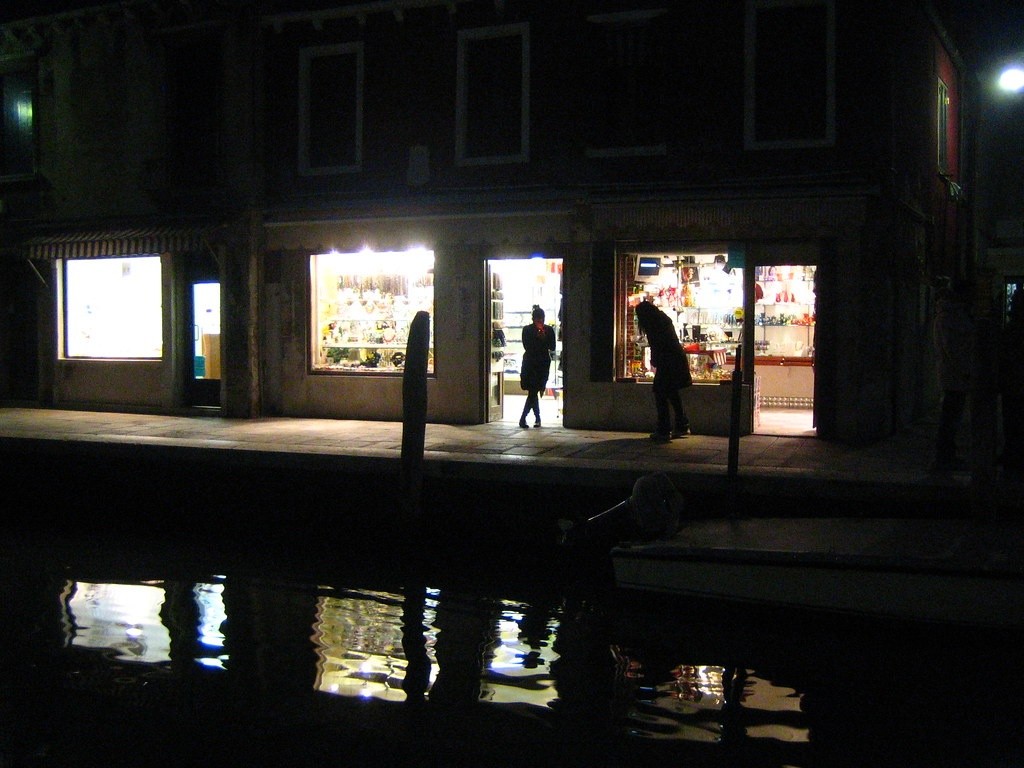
[518,305,556,427]
[636,301,693,440]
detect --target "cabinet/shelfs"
[503,311,532,342]
[676,268,817,328]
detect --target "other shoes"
[534,421,540,427]
[650,433,672,440]
[519,421,529,428]
[670,427,690,435]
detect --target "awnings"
[27,223,231,287]
[270,196,575,252]
